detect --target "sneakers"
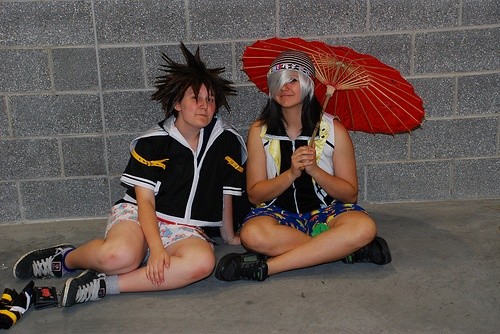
[215,251,268,282]
[12,243,76,279]
[344,236,392,266]
[60,268,106,307]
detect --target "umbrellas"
[240,36,425,136]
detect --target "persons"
[214,50,391,283]
[13,43,247,309]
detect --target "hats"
[266,51,315,83]
